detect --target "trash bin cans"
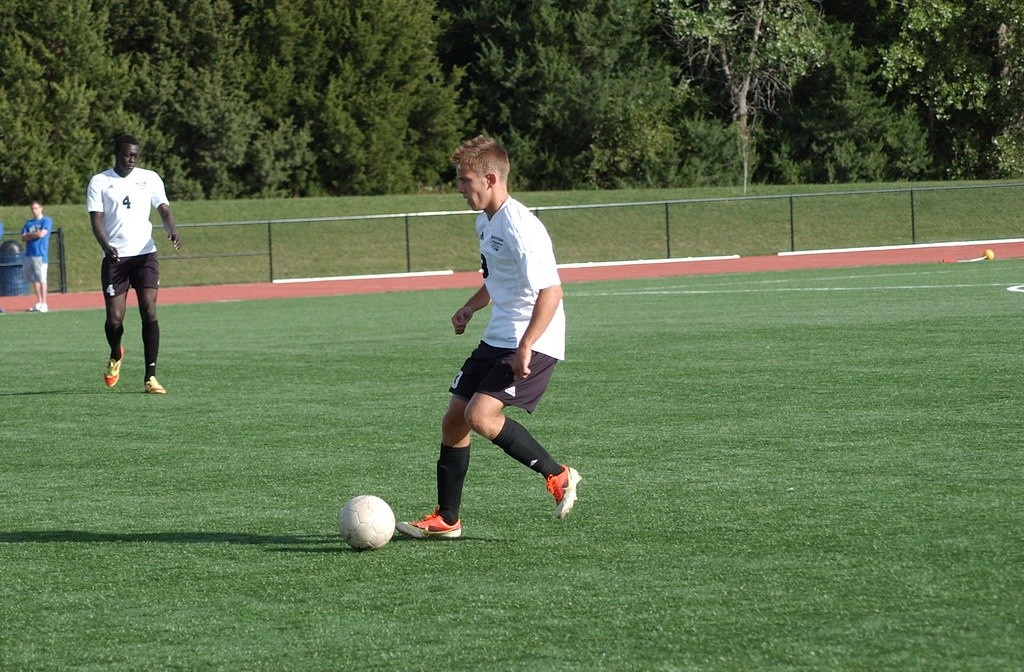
[0,239,29,297]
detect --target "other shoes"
[29,303,47,312]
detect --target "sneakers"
[104,344,124,387]
[395,506,461,538]
[546,464,582,518]
[144,376,166,393]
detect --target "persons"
[86,134,182,394]
[395,135,583,538]
[21,201,53,312]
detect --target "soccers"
[338,495,396,551]
